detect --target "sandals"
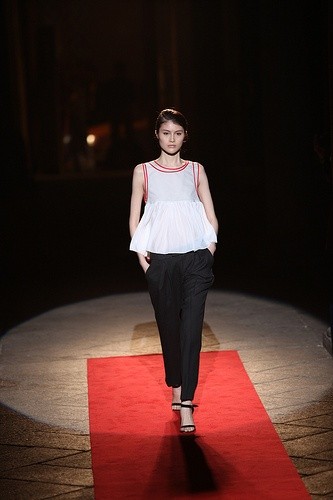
[180,403,198,433]
[171,402,182,412]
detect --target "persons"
[128,109,220,433]
